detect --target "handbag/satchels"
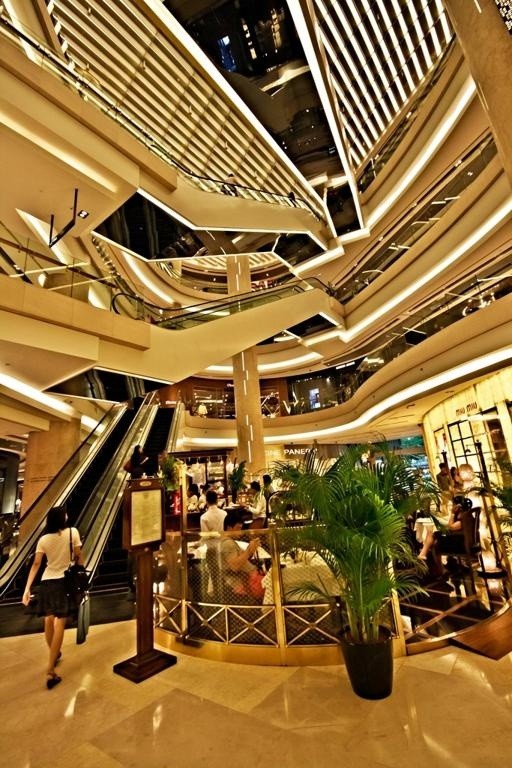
[124,460,130,473]
[76,596,91,643]
[63,565,88,600]
[248,571,267,600]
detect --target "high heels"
[46,671,62,690]
[54,652,62,667]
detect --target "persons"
[130,445,150,478]
[417,496,473,562]
[221,174,242,196]
[465,299,479,315]
[437,464,464,492]
[187,474,276,644]
[22,506,83,689]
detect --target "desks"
[266,547,390,642]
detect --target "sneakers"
[417,553,427,560]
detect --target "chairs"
[435,507,489,586]
[205,549,261,608]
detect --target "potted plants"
[262,430,430,699]
